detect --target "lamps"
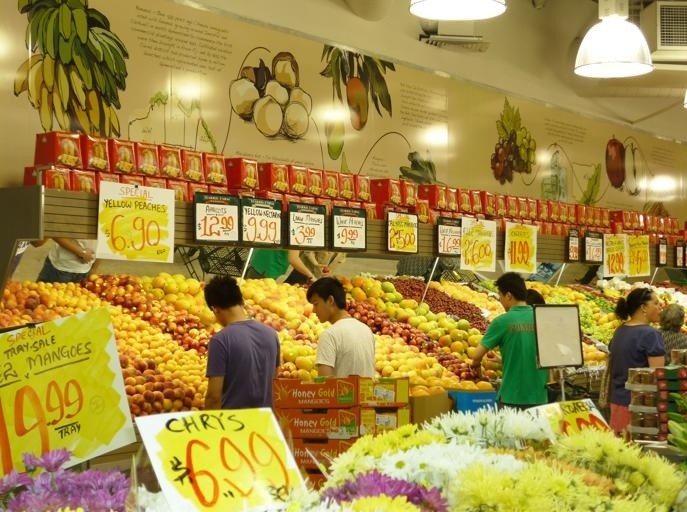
[573,1,653,78]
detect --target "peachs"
[229,52,312,139]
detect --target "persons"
[245,247,353,285]
[659,305,687,361]
[473,271,548,412]
[526,289,546,306]
[609,287,666,435]
[205,275,282,411]
[306,276,376,381]
[575,265,599,285]
[10,236,104,282]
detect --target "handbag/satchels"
[599,356,612,409]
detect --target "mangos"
[346,77,369,130]
[327,121,345,160]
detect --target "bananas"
[13,0,129,138]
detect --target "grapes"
[491,127,536,186]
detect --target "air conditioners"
[637,3,686,63]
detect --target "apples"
[606,135,642,195]
[0,271,503,422]
